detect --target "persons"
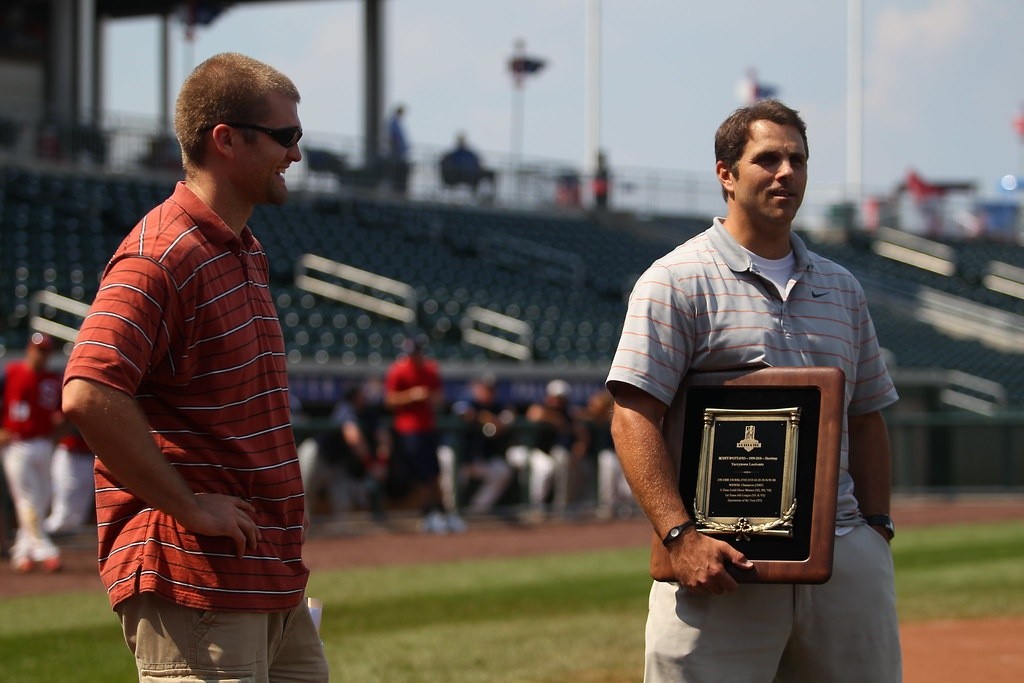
[387,99,411,199]
[588,149,614,208]
[39,396,97,535]
[1,330,65,570]
[887,163,944,239]
[446,133,507,208]
[57,53,329,682]
[603,99,908,683]
[289,331,643,520]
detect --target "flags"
[507,41,559,77]
[744,80,784,102]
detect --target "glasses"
[229,121,305,148]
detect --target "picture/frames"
[650,364,846,588]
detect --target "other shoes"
[12,539,63,570]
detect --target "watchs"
[663,521,693,545]
[862,514,896,541]
